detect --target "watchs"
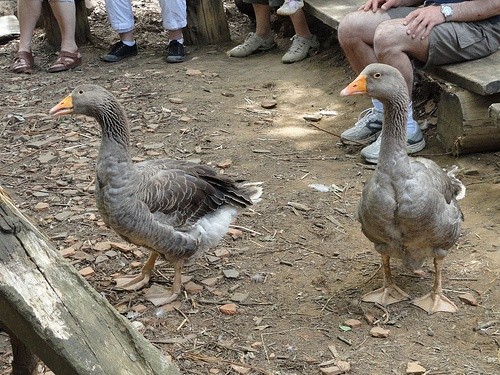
[440,3,453,22]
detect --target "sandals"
[12,46,34,74]
[47,48,81,73]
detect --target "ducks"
[339,62,468,315]
[47,83,264,307]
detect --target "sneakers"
[340,107,383,145]
[277,0,304,15]
[230,32,274,57]
[102,39,138,61]
[164,40,186,62]
[361,120,426,166]
[282,34,320,64]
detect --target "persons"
[12,0,81,73]
[99,0,187,62]
[230,0,320,63]
[340,0,500,163]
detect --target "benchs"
[303,0,500,156]
